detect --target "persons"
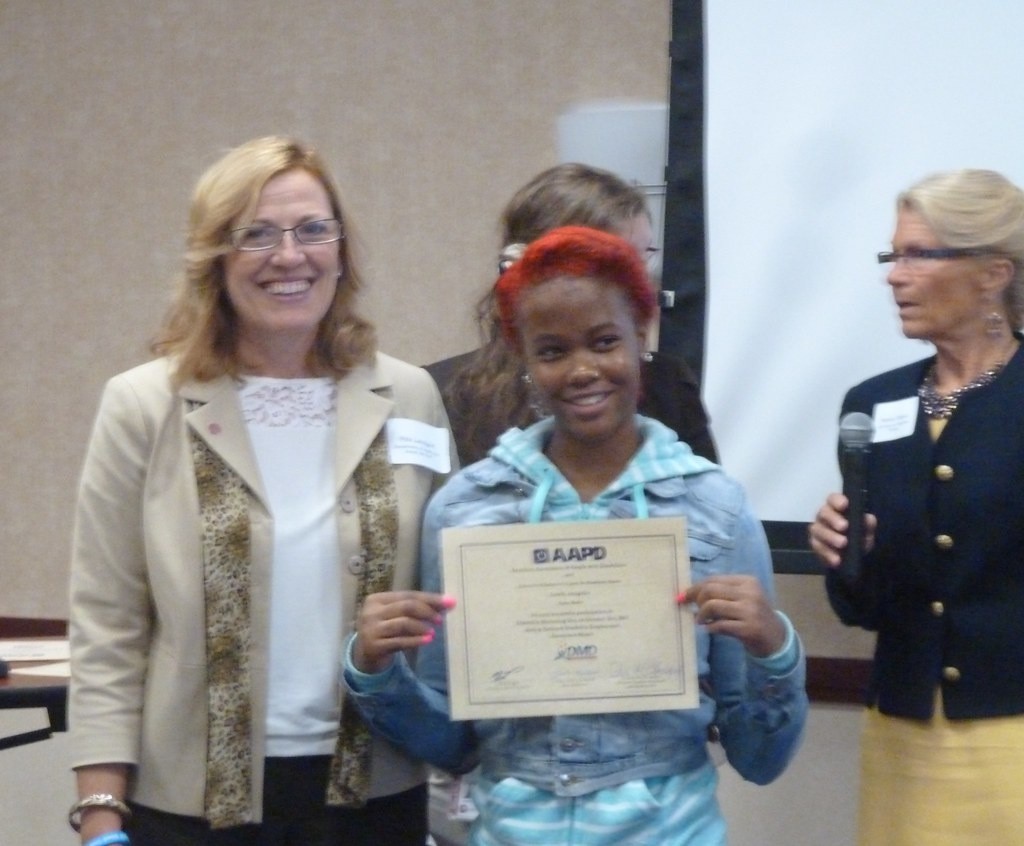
[420,161,721,470]
[340,227,808,846]
[808,168,1024,846]
[67,137,463,846]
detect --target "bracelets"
[68,794,132,832]
[84,830,132,846]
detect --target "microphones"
[837,410,875,579]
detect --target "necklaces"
[918,357,1002,418]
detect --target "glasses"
[227,217,343,252]
[877,247,984,275]
